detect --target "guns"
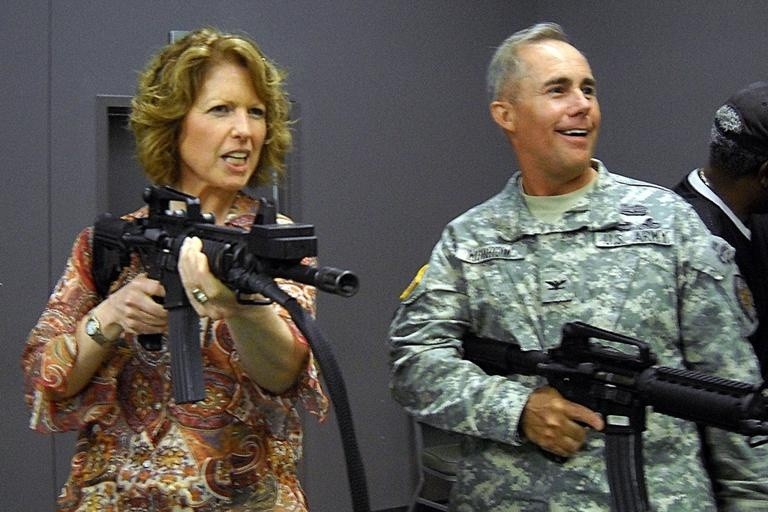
[91,188,359,404]
[460,323,768,510]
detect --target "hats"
[715,81,767,154]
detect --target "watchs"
[86,310,115,349]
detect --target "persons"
[669,76,766,383]
[21,29,328,512]
[386,21,767,512]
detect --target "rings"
[192,288,209,305]
